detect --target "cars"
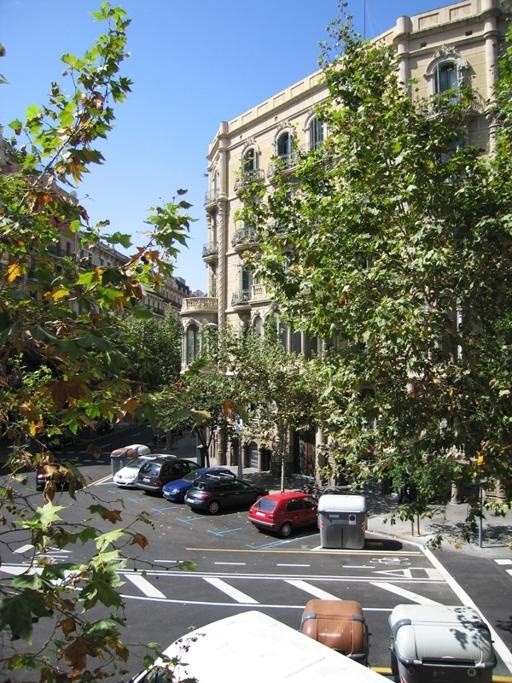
[248,491,317,537]
[112,454,201,494]
[162,467,269,514]
